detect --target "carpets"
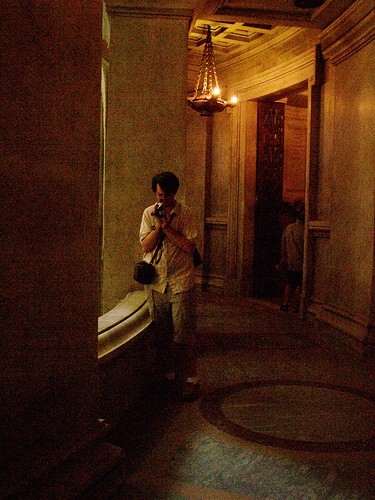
[165,431,347,500]
[201,330,317,354]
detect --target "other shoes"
[181,377,199,399]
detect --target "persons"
[277,204,304,312]
[139,172,201,401]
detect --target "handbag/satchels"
[133,260,156,284]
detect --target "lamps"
[186,24,238,117]
